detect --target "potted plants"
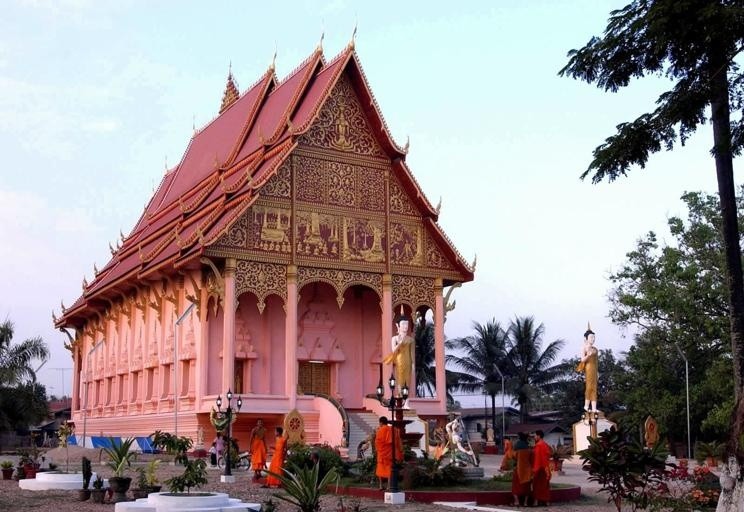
[223,446,241,472]
[0,434,163,503]
[698,439,726,467]
[549,443,574,471]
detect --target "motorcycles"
[218,450,251,471]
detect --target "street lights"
[376,374,409,492]
[216,388,242,475]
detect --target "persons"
[209,432,240,467]
[249,418,267,479]
[38,451,50,469]
[486,425,494,441]
[500,431,553,507]
[356,435,372,458]
[445,418,474,466]
[374,416,403,491]
[580,330,602,413]
[175,448,187,464]
[261,427,287,488]
[391,315,416,398]
